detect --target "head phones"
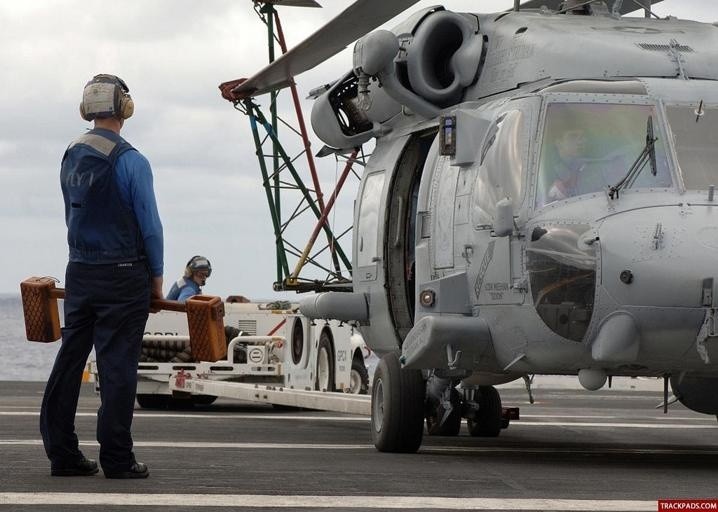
[185,255,211,278]
[79,74,134,121]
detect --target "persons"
[545,120,596,201]
[166,256,212,301]
[40,73,163,478]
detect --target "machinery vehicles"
[19,273,373,411]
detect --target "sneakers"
[51,456,99,477]
[100,462,149,479]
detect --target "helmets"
[83,74,129,118]
[189,256,209,272]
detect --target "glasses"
[195,270,210,278]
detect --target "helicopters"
[219,0,715,457]
